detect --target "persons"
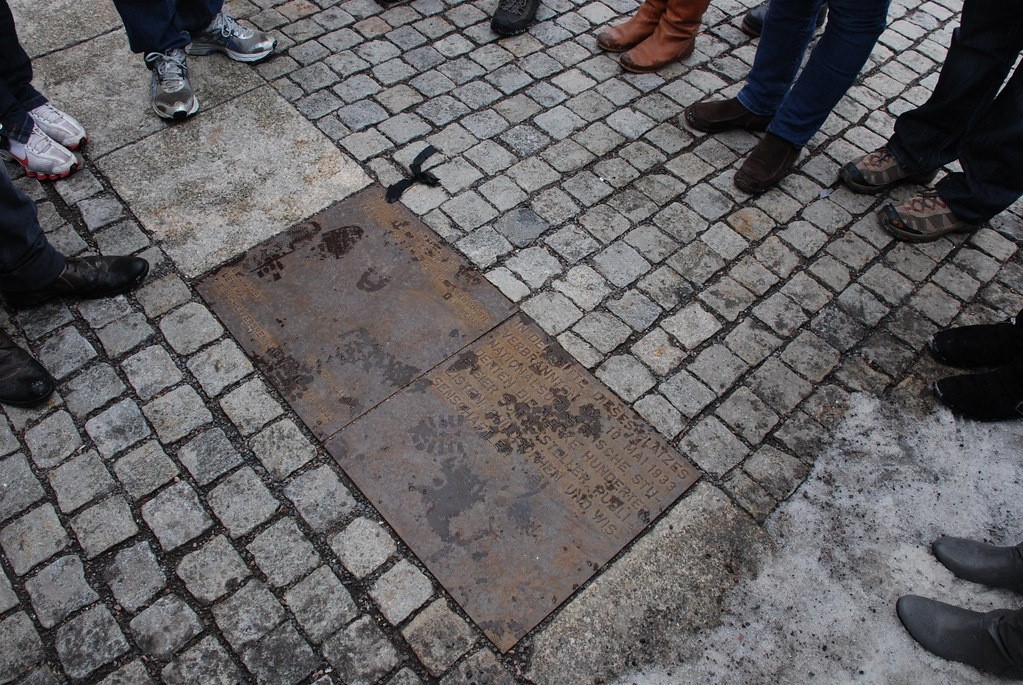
[0,159,149,408]
[490,0,541,35]
[0,0,88,180]
[840,0,1023,243]
[597,0,713,74]
[928,308,1023,424]
[112,0,278,120]
[895,536,1023,682]
[684,0,893,195]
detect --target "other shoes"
[686,96,774,134]
[735,130,801,193]
[931,308,1023,369]
[933,363,1023,422]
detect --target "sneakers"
[146,47,199,120]
[840,145,941,195]
[491,0,541,35]
[25,100,88,151]
[185,4,277,62]
[0,123,81,181]
[879,187,978,243]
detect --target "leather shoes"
[2,254,149,307]
[0,327,54,407]
[742,0,828,36]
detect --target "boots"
[934,537,1023,594]
[597,0,668,52]
[619,0,711,73]
[894,595,1023,681]
